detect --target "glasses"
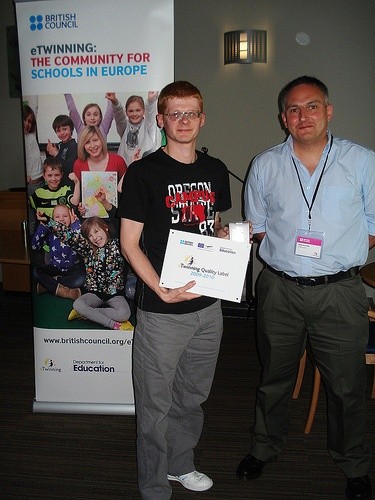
[163,110,202,121]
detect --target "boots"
[36,282,48,296]
[55,282,81,301]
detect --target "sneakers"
[67,308,88,321]
[120,321,134,330]
[167,470,213,491]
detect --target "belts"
[266,265,360,287]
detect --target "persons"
[234,75,375,500]
[118,82,253,500]
[21,95,163,331]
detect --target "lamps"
[223,30,269,65]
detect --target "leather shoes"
[236,453,266,480]
[346,473,372,500]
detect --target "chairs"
[293,297,375,434]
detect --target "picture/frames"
[81,171,118,218]
